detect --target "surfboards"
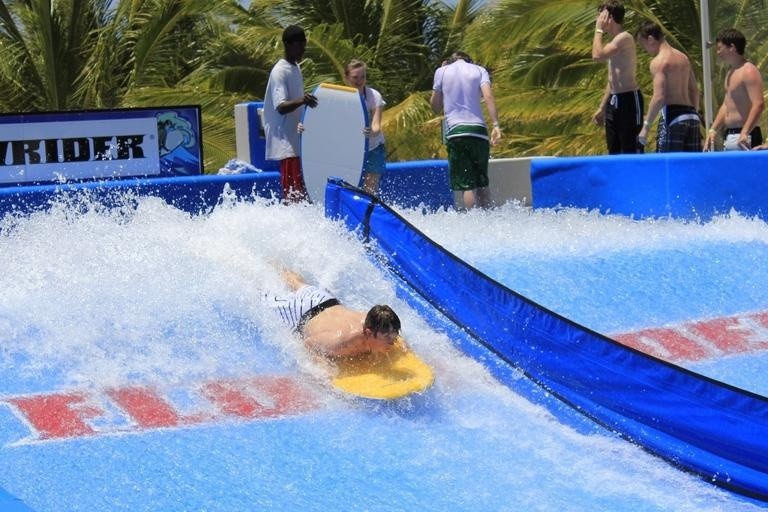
[299,83,370,208]
[263,268,434,399]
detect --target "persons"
[260,22,319,205]
[254,251,402,362]
[296,55,389,196]
[700,26,764,151]
[590,0,645,156]
[430,50,504,212]
[752,143,768,151]
[633,20,703,155]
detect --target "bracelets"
[594,29,604,34]
[708,127,716,131]
[643,121,653,128]
[492,124,500,130]
